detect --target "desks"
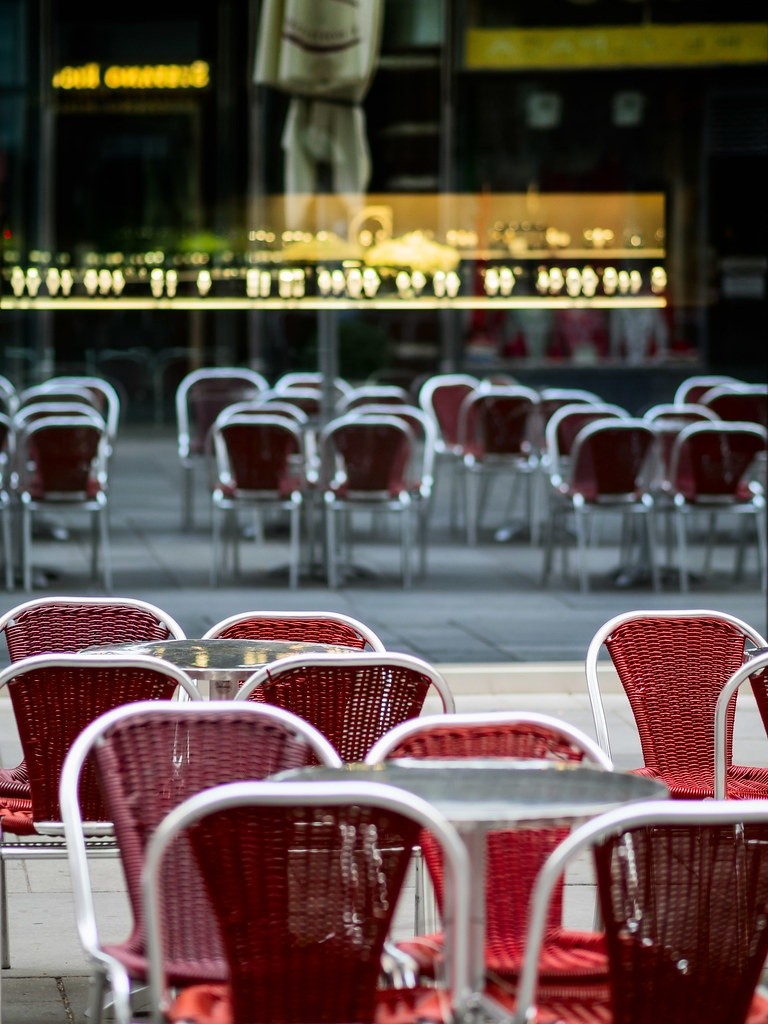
[78,635,368,1023]
[264,755,674,1024]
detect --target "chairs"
[176,364,768,595]
[0,595,768,1024]
[0,367,121,597]
